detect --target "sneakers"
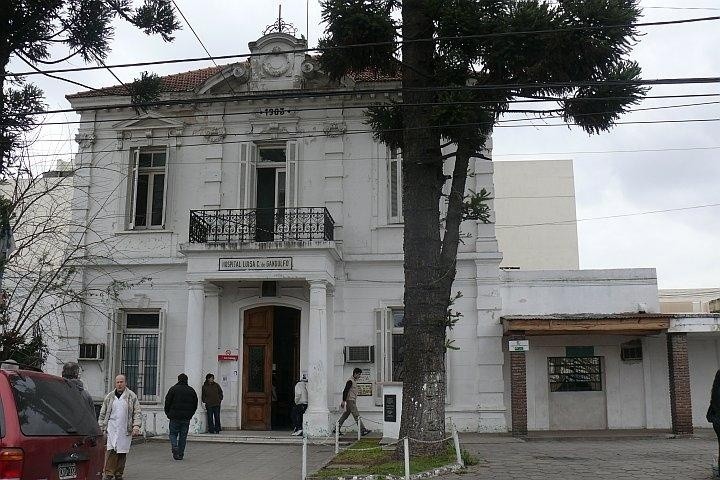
[361,429,372,436]
[172,447,183,460]
[333,429,343,436]
[104,475,123,480]
[291,429,303,436]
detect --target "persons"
[331,367,372,436]
[165,373,198,461]
[706,367,720,478]
[97,374,142,480]
[291,377,308,437]
[62,361,97,417]
[200,373,223,434]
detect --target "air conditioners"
[621,342,643,361]
[344,345,374,363]
[79,343,105,361]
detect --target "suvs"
[0,363,106,479]
